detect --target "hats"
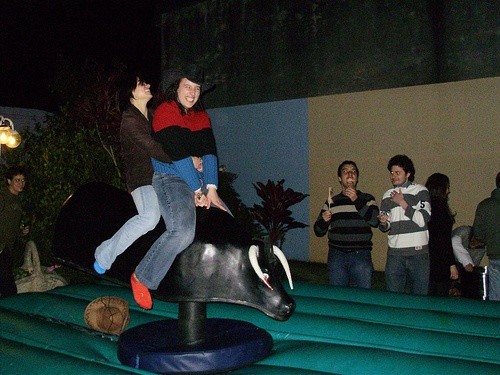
[181,64,205,85]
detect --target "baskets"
[14,241,69,294]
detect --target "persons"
[0,166,29,299]
[377,155,432,297]
[314,161,379,287]
[91,72,162,276]
[425,170,500,302]
[130,72,227,309]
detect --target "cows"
[51,181,297,322]
